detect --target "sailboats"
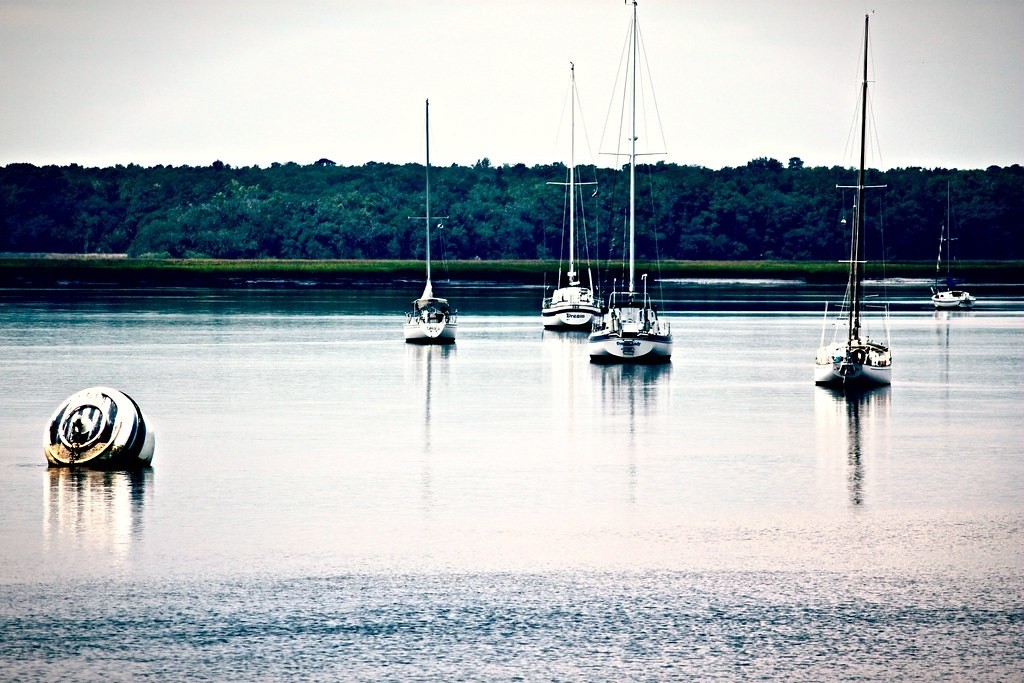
[584,2,671,363]
[930,180,976,312]
[814,14,892,391]
[538,62,602,330]
[404,95,462,344]
